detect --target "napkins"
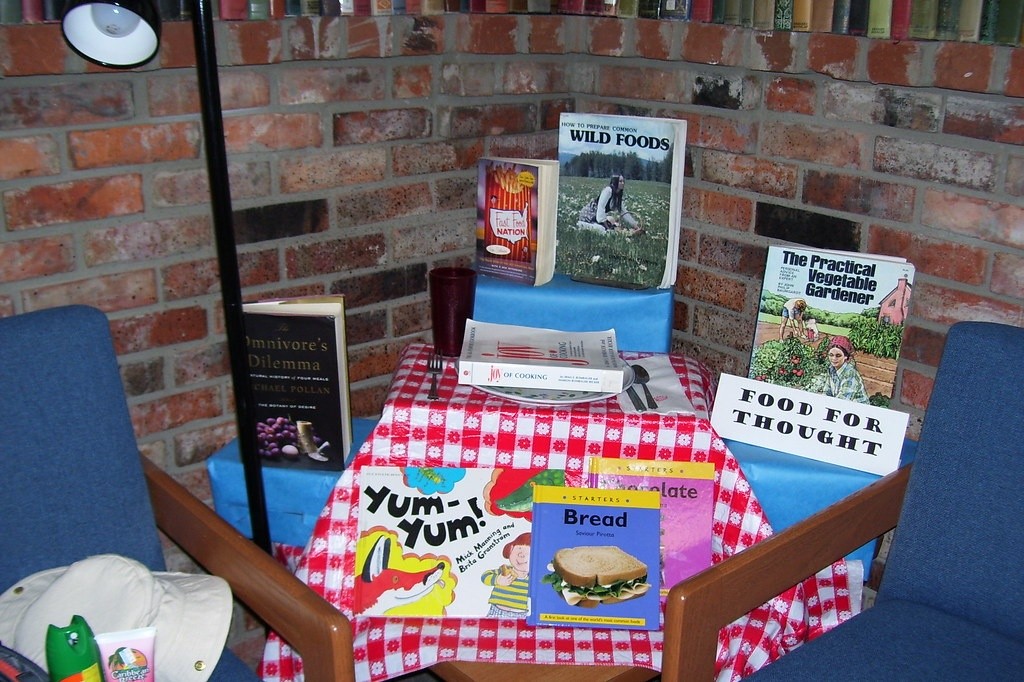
[618,353,697,416]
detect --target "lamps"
[61,0,272,557]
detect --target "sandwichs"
[540,546,652,608]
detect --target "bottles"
[45,615,104,682]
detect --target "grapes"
[257,417,322,459]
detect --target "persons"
[579,173,644,231]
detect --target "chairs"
[0,304,357,682]
[661,321,1024,682]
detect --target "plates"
[454,355,635,407]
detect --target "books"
[354,464,542,621]
[553,112,688,289]
[457,318,625,395]
[748,243,916,409]
[526,482,662,631]
[475,155,560,287]
[242,295,354,472]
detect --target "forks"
[427,348,443,399]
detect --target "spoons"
[631,365,658,410]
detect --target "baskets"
[618,212,644,238]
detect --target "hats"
[0,553,233,682]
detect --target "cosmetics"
[95,625,158,682]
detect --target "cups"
[427,266,478,357]
